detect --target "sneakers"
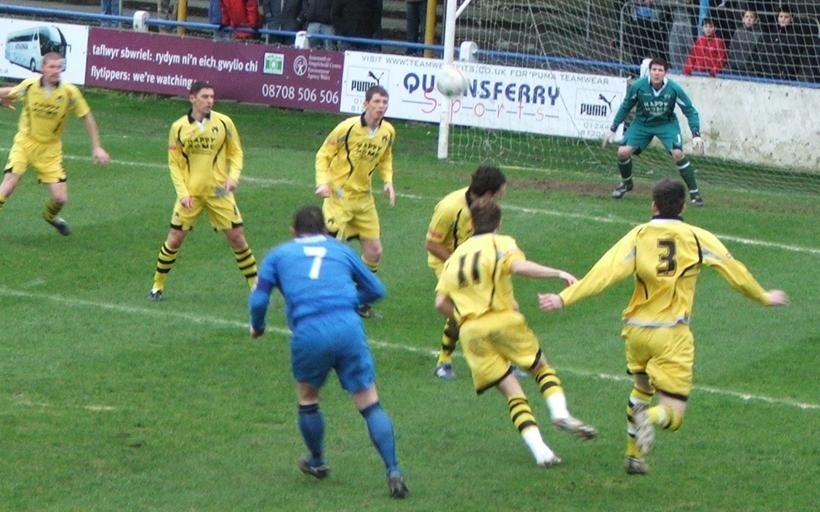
[511,365,526,377]
[634,413,655,453]
[149,283,165,299]
[43,212,69,234]
[553,417,595,439]
[388,471,408,498]
[689,192,704,206]
[626,457,647,474]
[357,305,375,317]
[298,460,329,479]
[536,455,561,468]
[434,362,455,379]
[612,181,633,198]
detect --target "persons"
[249,206,409,499]
[436,199,599,468]
[0,52,112,236]
[728,9,768,75]
[315,86,397,320]
[149,82,259,302]
[427,166,529,378]
[537,180,790,474]
[747,6,805,82]
[214,0,261,43]
[304,0,383,52]
[406,0,428,55]
[612,0,678,81]
[603,59,704,206]
[263,0,310,50]
[682,18,727,78]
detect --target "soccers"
[437,67,466,96]
